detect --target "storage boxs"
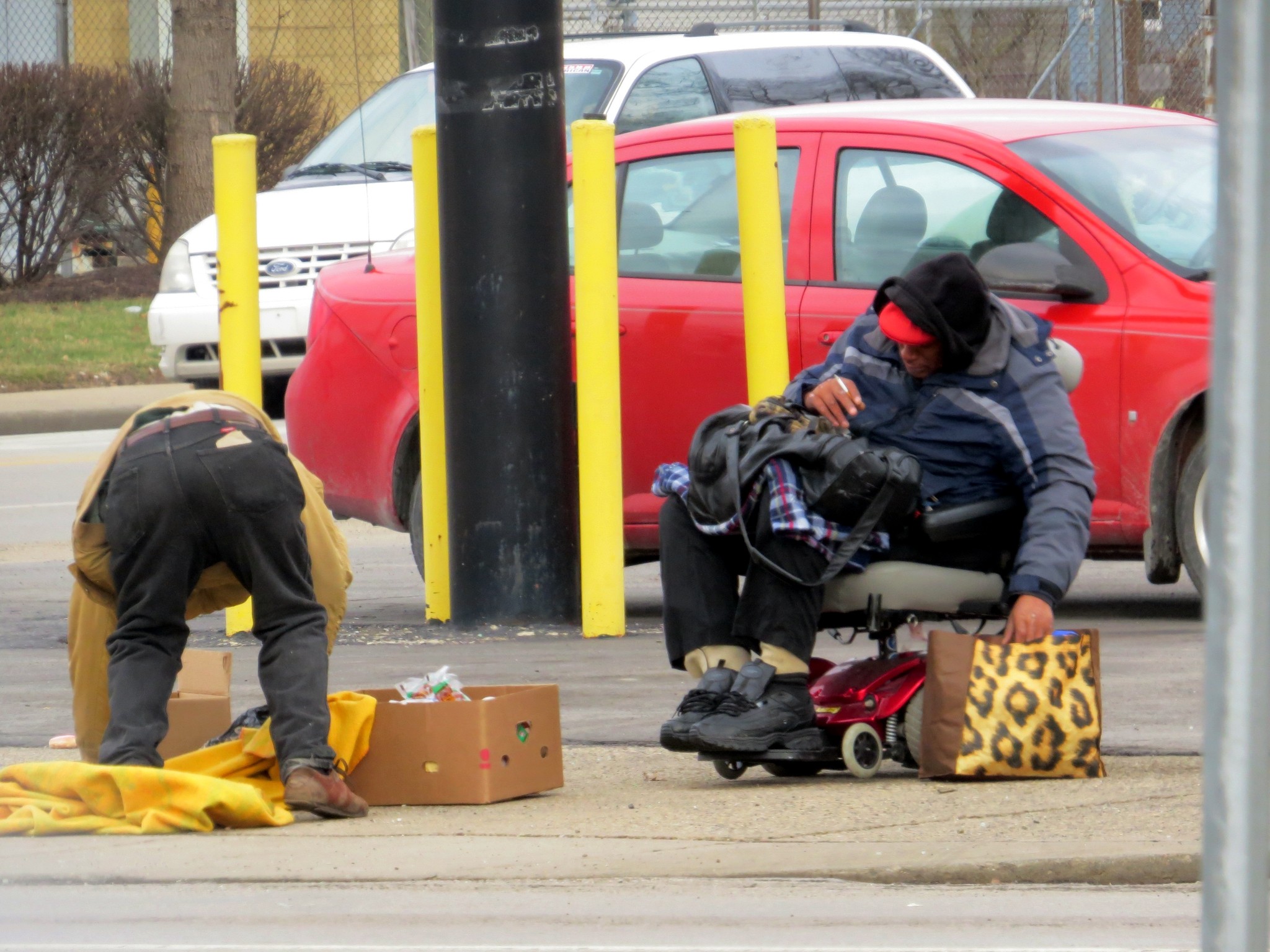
[328,686,564,810]
[157,650,233,759]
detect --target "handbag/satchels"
[922,612,1105,781]
[686,401,924,586]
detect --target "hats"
[880,302,931,345]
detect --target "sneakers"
[692,659,824,752]
[660,666,738,751]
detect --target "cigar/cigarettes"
[834,375,853,401]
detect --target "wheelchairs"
[690,342,1085,782]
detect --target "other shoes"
[284,759,370,818]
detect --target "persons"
[65,388,369,822]
[658,253,1096,754]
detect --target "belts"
[128,410,259,446]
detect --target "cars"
[282,95,1211,613]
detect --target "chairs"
[611,206,672,280]
[847,182,928,286]
[970,186,1065,266]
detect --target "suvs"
[146,18,990,424]
